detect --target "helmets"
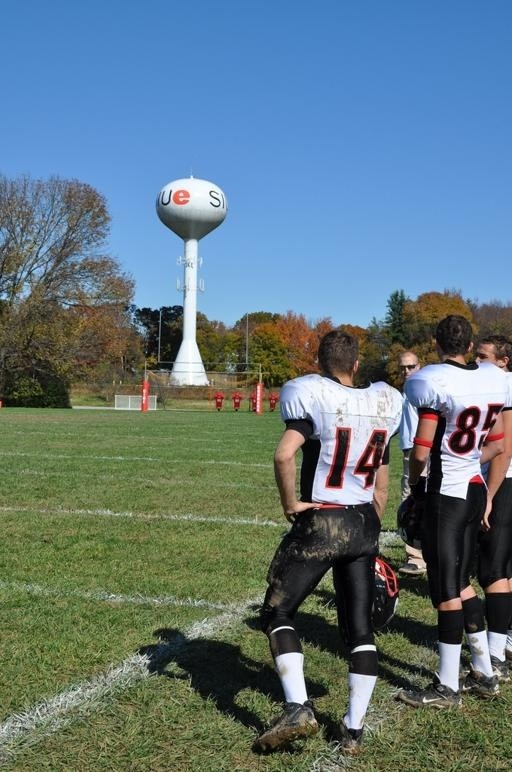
[397,495,426,550]
[372,557,401,633]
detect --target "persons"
[214,386,278,413]
[252,331,404,757]
[397,348,431,577]
[397,314,510,709]
[475,331,511,687]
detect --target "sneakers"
[397,655,511,710]
[253,701,319,756]
[334,709,364,755]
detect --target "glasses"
[397,363,418,371]
